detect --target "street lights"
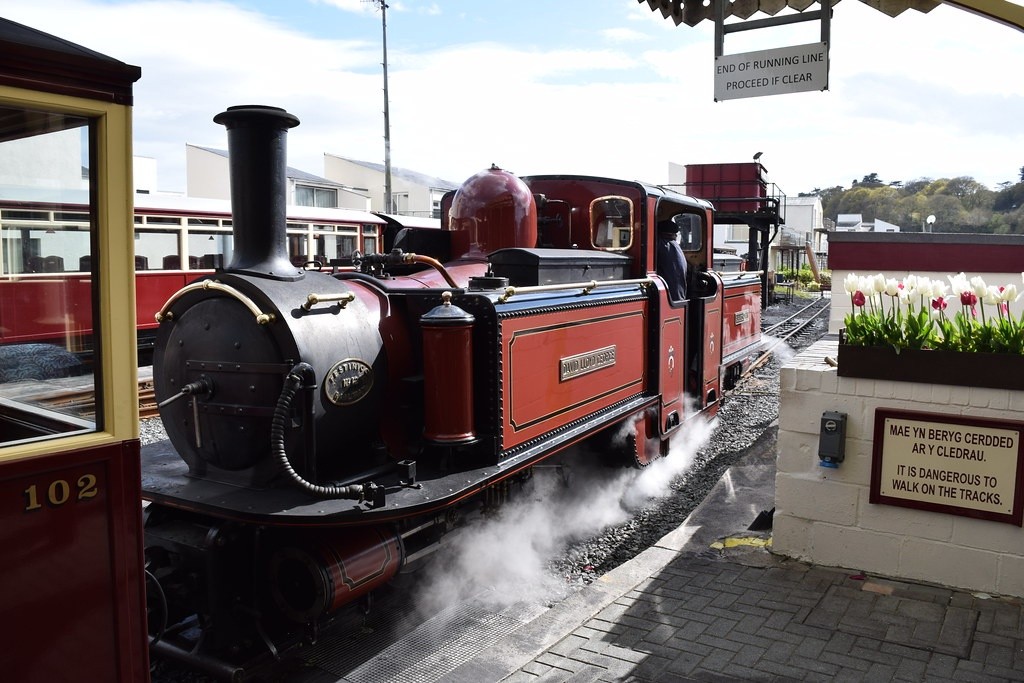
[926,214,936,233]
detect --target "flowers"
[841,270,1024,355]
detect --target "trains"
[1,185,441,357]
[140,104,763,682]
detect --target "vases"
[837,329,1024,391]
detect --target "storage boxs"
[684,163,768,213]
